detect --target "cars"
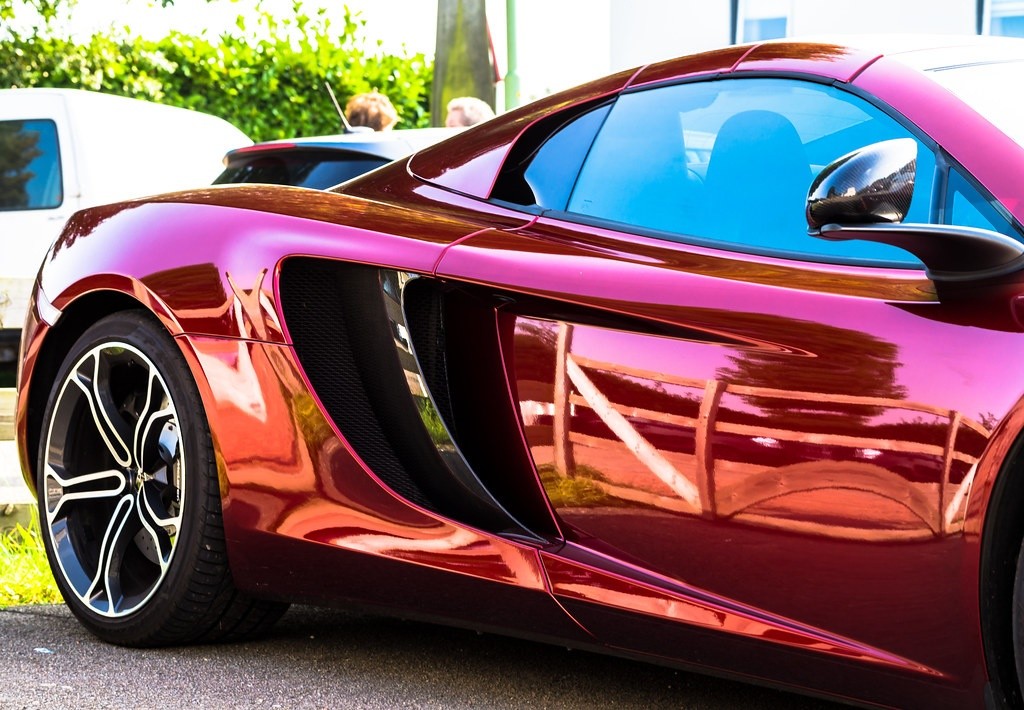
[0,90,256,384]
[15,39,1024,710]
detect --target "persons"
[339,91,498,136]
